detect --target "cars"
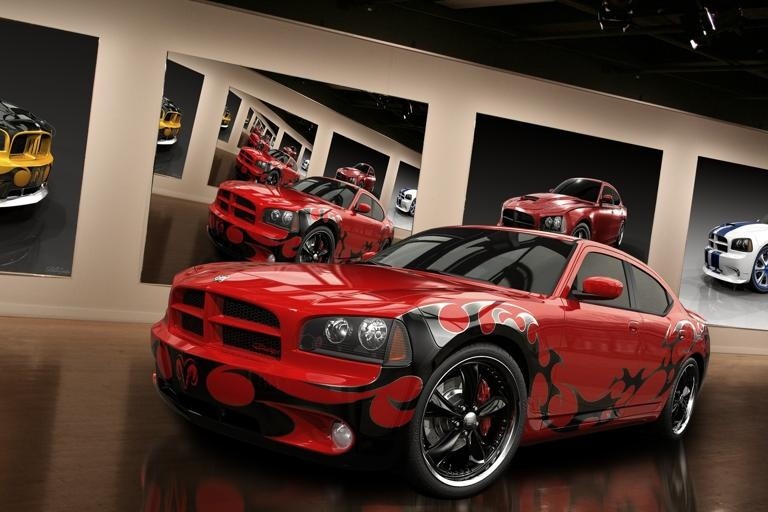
[0,96,55,207]
[703,216,768,294]
[157,96,181,145]
[396,187,417,216]
[206,104,394,264]
[151,225,710,499]
[497,177,627,249]
